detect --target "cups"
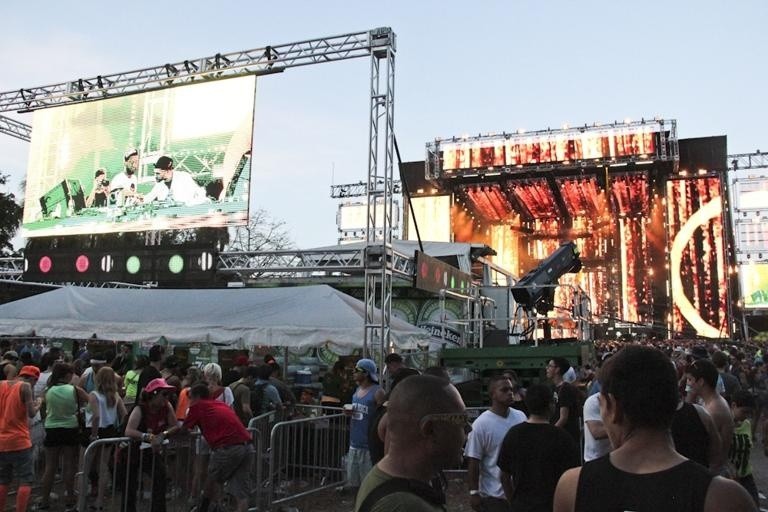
[345,404,352,416]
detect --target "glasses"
[691,361,699,372]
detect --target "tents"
[0,285,456,357]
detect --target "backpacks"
[251,383,268,410]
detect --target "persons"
[84,167,112,208]
[2,337,766,511]
[569,285,585,339]
[131,154,212,210]
[107,147,145,208]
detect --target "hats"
[19,365,40,378]
[357,359,378,382]
[144,378,177,392]
[124,149,138,161]
[154,156,174,170]
